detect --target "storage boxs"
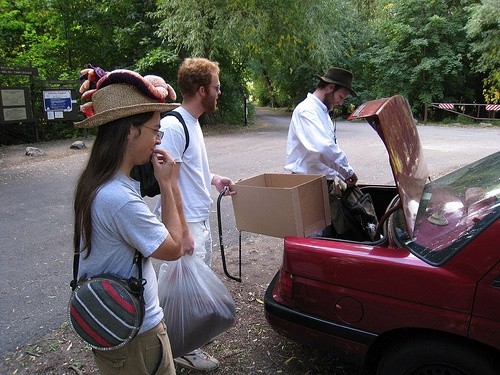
[228,172,331,239]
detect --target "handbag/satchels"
[339,180,381,241]
[154,251,238,361]
[65,272,147,354]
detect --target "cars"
[264,94,500,375]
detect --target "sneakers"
[173,347,222,372]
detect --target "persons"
[66,64,194,375]
[284,67,358,237]
[156,57,237,370]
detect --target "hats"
[314,66,357,95]
[71,61,183,131]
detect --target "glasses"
[137,121,167,141]
[213,83,220,91]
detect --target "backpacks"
[129,109,190,198]
